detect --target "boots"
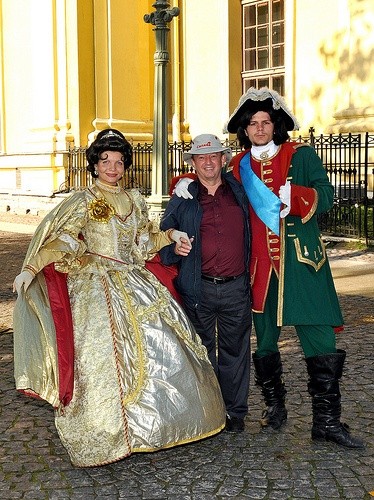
[251,351,288,430]
[302,349,366,448]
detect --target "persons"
[158,134,253,433]
[12,129,227,467]
[168,86,367,450]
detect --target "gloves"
[12,271,33,293]
[172,177,195,200]
[170,229,193,250]
[279,180,291,218]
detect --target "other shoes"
[226,414,245,432]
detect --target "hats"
[224,85,302,132]
[183,134,232,169]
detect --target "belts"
[201,270,247,285]
